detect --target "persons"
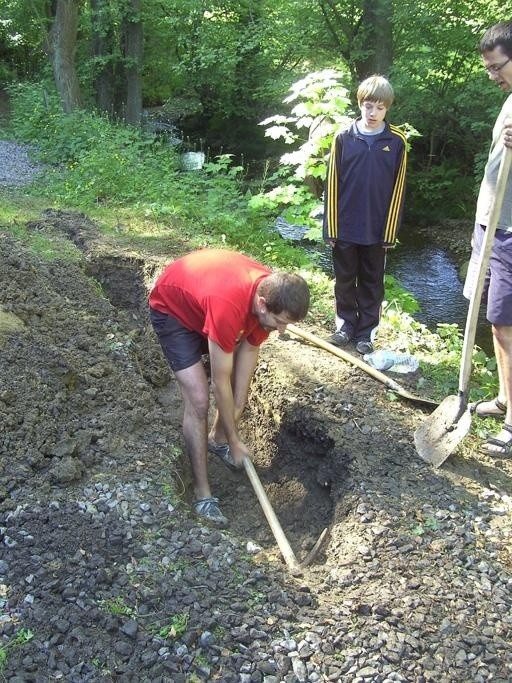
[147,248,311,531]
[463,18,512,458]
[320,76,407,354]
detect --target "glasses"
[484,59,510,73]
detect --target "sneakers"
[328,329,351,345]
[356,341,373,355]
[208,431,243,472]
[192,495,229,529]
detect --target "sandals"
[479,424,512,459]
[468,396,506,420]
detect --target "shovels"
[284,320,442,407]
[413,146,512,468]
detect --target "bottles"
[363,350,419,373]
